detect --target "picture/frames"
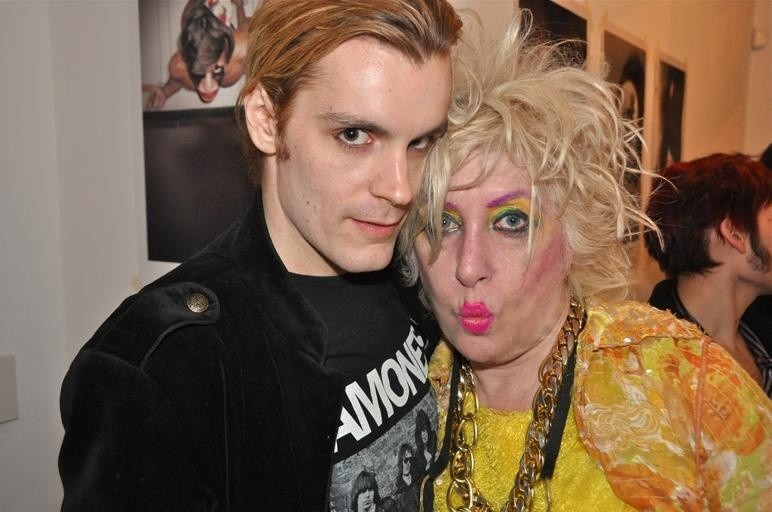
[649,44,692,193]
[596,15,650,250]
[509,2,596,92]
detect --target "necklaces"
[443,287,587,511]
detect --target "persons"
[350,470,385,512]
[399,10,772,511]
[396,442,415,491]
[54,1,464,511]
[415,408,437,474]
[643,151,772,401]
[142,0,252,110]
